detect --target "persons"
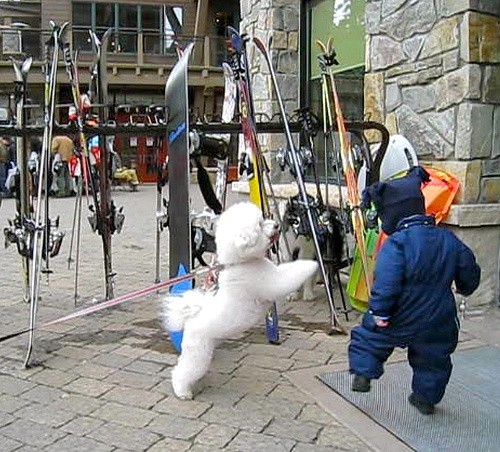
[347,166,481,415]
[0,136,74,198]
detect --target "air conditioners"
[152,201,320,394]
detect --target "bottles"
[362,166,431,235]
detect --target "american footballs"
[207,60,240,268]
[158,39,210,301]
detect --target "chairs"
[352,373,369,392]
[408,393,434,414]
